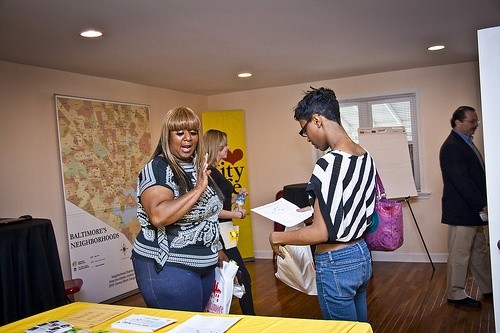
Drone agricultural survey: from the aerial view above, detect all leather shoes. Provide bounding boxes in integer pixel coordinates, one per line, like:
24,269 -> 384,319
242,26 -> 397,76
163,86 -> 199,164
447,296 -> 482,307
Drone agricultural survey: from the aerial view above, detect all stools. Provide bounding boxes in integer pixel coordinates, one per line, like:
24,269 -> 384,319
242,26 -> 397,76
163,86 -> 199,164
63,278 -> 83,303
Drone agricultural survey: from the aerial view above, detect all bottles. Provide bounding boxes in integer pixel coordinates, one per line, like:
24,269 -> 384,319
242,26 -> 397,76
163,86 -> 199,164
235,193 -> 246,212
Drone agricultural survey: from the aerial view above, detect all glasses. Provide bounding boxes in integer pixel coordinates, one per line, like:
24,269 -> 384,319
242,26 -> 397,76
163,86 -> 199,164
299,112 -> 321,137
464,119 -> 480,123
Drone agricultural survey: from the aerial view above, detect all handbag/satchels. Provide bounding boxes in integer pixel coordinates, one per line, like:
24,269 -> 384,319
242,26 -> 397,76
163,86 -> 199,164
274,222 -> 318,295
218,220 -> 239,248
360,170 -> 405,251
203,259 -> 239,314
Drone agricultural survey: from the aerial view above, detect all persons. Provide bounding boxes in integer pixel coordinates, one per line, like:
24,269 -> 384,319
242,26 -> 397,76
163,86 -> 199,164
439,105 -> 493,308
130,105 -> 230,313
269,86 -> 378,323
197,129 -> 257,316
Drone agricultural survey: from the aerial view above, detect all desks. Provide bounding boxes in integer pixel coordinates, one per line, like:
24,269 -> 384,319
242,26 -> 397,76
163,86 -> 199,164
0,301 -> 373,333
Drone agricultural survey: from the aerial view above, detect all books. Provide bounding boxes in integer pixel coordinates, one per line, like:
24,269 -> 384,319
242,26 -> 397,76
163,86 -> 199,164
110,314 -> 177,332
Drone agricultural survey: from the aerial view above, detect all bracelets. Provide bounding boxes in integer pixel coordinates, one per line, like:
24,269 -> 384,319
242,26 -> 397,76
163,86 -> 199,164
239,210 -> 243,219
